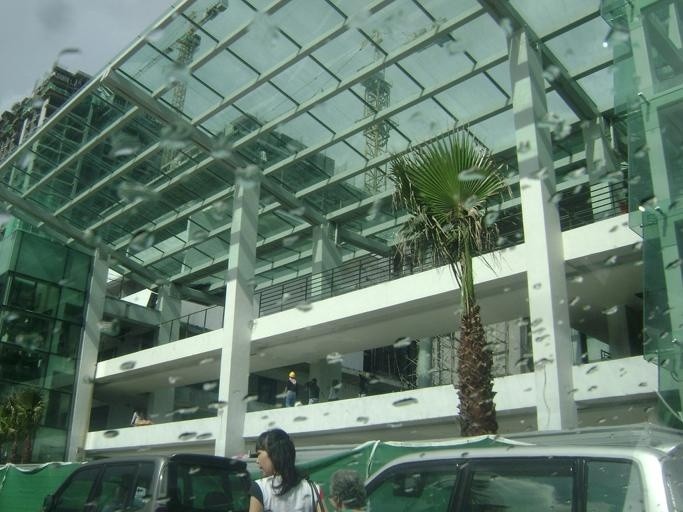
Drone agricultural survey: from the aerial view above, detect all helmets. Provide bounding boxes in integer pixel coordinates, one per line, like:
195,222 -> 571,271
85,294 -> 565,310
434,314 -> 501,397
289,371 -> 296,378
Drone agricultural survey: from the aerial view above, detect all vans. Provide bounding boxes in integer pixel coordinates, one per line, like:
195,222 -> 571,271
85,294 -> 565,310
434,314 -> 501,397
41,451 -> 251,512
358,445 -> 680,512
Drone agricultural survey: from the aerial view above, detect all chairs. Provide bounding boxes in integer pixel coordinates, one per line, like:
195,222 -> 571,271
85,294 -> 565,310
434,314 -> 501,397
203,492 -> 227,512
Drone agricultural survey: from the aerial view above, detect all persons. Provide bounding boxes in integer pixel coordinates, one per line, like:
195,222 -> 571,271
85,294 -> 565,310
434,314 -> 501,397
303,377 -> 320,404
247,429 -> 328,512
327,468 -> 368,512
327,379 -> 340,401
285,370 -> 298,407
131,406 -> 144,426
